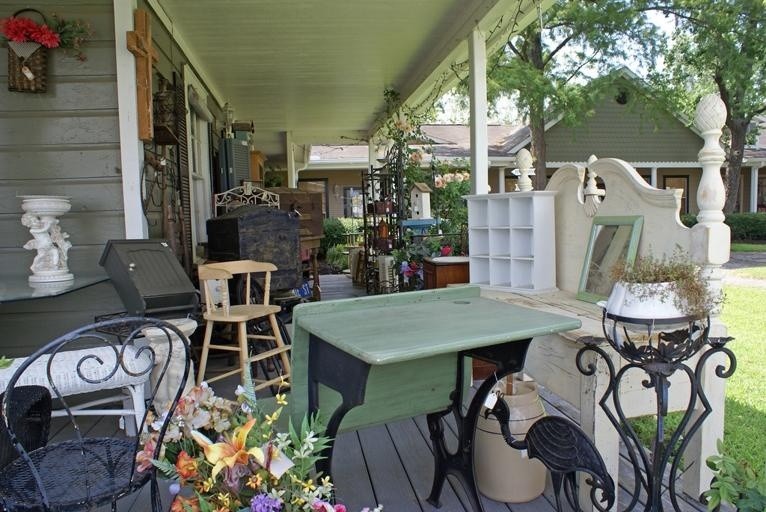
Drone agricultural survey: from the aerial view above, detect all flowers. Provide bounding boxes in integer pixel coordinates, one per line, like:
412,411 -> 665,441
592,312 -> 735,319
0,16 -> 99,65
129,344 -> 387,512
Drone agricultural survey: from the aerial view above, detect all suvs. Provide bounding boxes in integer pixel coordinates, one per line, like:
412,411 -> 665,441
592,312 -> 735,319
474,380 -> 548,503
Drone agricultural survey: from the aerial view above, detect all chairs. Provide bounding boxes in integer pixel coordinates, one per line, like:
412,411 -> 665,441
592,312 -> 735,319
0,317 -> 191,511
196,259 -> 292,412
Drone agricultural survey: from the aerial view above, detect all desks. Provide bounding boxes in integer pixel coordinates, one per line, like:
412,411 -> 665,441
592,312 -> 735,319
289,283 -> 583,511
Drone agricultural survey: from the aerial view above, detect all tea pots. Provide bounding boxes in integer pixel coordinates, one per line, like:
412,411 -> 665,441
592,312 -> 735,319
576,214 -> 645,305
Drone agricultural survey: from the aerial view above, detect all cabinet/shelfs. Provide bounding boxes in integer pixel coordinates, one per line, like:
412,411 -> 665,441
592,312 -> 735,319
460,190 -> 562,296
421,257 -> 469,289
361,164 -> 403,295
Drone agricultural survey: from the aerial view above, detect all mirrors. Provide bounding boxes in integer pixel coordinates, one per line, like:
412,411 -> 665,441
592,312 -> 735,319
576,214 -> 645,305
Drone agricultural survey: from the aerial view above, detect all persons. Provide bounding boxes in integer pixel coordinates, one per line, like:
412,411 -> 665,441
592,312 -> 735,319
21,212 -> 59,272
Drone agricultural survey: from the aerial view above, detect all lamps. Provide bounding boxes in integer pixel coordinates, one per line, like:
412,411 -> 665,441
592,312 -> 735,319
196,259 -> 292,412
0,317 -> 191,511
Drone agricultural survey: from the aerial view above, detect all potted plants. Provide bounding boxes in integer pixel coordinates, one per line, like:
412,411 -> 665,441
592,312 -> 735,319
608,240 -> 731,335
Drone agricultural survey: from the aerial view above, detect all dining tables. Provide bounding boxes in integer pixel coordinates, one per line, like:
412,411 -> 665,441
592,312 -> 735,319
608,240 -> 731,335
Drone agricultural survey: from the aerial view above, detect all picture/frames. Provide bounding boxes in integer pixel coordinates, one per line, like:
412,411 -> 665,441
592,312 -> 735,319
289,283 -> 583,511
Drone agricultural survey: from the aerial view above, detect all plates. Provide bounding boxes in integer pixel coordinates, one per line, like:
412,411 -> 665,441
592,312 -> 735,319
421,257 -> 469,289
361,164 -> 403,295
460,190 -> 562,296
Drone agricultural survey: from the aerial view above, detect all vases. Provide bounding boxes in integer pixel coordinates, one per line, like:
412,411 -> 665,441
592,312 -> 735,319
0,16 -> 99,65
